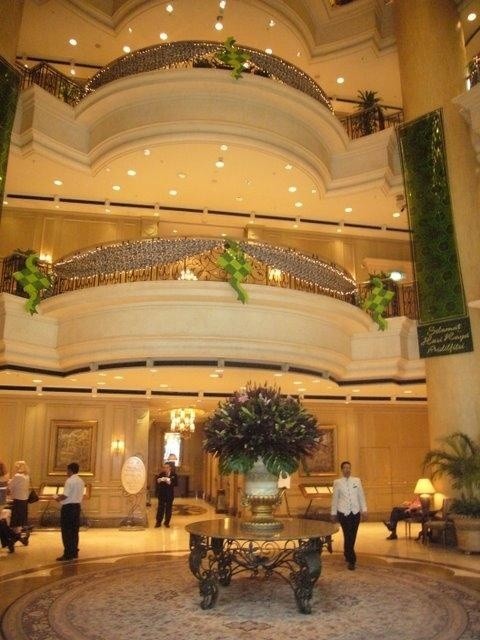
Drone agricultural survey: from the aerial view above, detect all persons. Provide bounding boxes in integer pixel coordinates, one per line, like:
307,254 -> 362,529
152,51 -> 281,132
0,462 -> 10,487
4,460 -> 32,531
329,461 -> 368,571
52,463 -> 85,561
382,496 -> 424,541
153,461 -> 178,528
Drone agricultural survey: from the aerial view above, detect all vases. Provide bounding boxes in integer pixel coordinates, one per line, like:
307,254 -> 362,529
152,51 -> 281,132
237,455 -> 285,534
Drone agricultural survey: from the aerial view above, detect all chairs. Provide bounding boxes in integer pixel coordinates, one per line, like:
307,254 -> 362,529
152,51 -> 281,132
402,491 -> 445,541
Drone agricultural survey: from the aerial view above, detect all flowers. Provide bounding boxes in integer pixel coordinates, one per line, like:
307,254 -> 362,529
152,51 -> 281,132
199,377 -> 326,482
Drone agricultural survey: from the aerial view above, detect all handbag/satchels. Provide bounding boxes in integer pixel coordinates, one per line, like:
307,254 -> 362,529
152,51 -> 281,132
27,491 -> 40,503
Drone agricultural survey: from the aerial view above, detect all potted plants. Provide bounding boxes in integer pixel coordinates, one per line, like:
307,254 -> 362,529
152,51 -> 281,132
420,430 -> 480,556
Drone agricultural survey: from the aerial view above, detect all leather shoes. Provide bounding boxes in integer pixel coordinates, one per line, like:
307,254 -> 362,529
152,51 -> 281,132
73,552 -> 78,558
56,554 -> 73,561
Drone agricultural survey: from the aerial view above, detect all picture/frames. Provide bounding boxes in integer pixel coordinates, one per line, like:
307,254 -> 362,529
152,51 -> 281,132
295,422 -> 342,479
47,417 -> 99,478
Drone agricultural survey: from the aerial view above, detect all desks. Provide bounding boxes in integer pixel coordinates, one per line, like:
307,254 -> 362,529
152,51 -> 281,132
418,516 -> 458,549
184,513 -> 342,615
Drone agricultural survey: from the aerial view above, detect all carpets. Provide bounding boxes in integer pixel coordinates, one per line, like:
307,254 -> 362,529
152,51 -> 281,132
0,554 -> 480,640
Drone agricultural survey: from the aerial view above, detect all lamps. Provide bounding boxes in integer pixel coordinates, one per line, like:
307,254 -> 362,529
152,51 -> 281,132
168,407 -> 198,441
412,479 -> 442,521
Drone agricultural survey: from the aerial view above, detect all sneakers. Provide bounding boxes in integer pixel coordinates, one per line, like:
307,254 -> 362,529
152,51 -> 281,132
155,521 -> 161,527
384,521 -> 394,530
345,552 -> 356,570
386,533 -> 397,539
23,532 -> 30,546
164,521 -> 169,527
